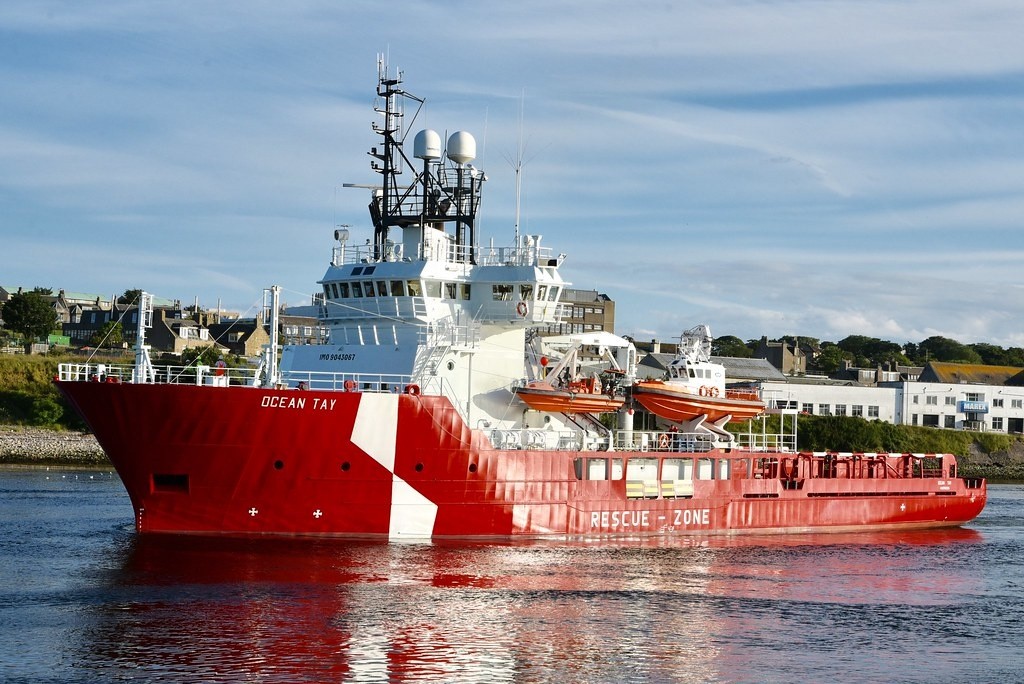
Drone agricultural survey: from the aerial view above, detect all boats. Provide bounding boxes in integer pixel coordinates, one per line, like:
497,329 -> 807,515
626,323 -> 768,425
513,340 -> 627,415
51,42 -> 989,543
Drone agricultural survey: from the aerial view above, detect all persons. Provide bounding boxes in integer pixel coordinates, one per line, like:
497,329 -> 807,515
183,359 -> 195,383
367,284 -> 415,297
667,424 -> 679,447
215,354 -> 227,377
194,356 -> 203,384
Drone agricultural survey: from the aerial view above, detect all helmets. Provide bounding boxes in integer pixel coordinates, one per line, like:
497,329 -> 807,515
219,355 -> 224,360
197,356 -> 201,360
186,359 -> 190,363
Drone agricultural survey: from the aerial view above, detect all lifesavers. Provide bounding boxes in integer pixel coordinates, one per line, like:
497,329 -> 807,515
659,434 -> 668,448
709,387 -> 719,398
699,386 -> 708,396
517,302 -> 528,317
